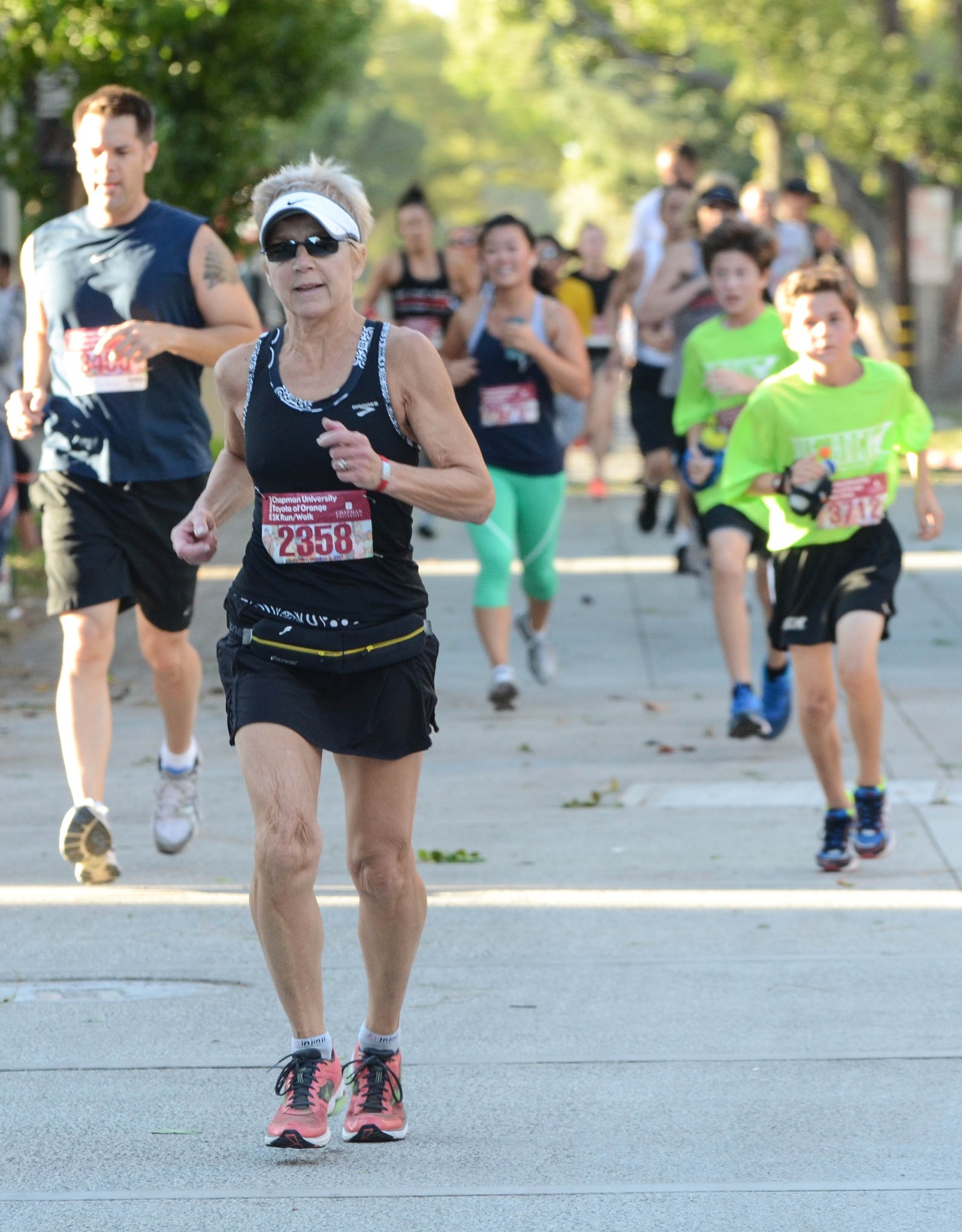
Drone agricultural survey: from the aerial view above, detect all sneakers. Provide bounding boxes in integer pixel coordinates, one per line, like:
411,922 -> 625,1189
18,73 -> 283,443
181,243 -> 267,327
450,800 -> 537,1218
729,686 -> 770,738
488,669 -> 519,711
153,753 -> 198,855
817,790 -> 857,868
517,612 -> 559,685
854,780 -> 889,857
60,803 -> 119,884
339,1044 -> 409,1142
760,659 -> 792,739
265,1047 -> 348,1150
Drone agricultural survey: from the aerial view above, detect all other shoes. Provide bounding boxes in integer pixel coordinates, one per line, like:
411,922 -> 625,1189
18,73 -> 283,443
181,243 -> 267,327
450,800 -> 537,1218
638,489 -> 660,530
588,480 -> 605,498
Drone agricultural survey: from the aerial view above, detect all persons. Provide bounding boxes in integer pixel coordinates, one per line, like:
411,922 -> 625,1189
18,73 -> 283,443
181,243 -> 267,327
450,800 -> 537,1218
4,84 -> 263,884
363,182 -> 472,538
170,151 -> 495,1147
569,221 -> 623,500
437,213 -> 592,710
637,182 -> 757,561
446,139 -> 867,575
0,213 -> 286,636
672,220 -> 798,741
719,262 -> 944,872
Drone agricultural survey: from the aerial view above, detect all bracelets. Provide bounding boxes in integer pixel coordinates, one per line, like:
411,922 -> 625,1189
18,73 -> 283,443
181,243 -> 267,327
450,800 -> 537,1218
375,454 -> 391,492
771,467 -> 793,496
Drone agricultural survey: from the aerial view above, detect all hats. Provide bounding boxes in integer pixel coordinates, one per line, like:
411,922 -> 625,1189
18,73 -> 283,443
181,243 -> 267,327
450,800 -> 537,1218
786,180 -> 821,206
694,186 -> 739,211
259,193 -> 361,247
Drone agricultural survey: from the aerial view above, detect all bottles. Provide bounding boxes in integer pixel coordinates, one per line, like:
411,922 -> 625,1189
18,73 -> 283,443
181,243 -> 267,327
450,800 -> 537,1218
789,448 -> 832,511
503,317 -> 525,370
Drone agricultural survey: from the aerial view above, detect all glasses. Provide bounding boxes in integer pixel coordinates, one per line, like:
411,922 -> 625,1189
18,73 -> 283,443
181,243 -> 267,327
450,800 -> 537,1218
262,236 -> 346,261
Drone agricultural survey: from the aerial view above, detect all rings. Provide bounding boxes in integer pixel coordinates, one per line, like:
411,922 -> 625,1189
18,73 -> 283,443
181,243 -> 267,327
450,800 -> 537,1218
338,457 -> 346,470
